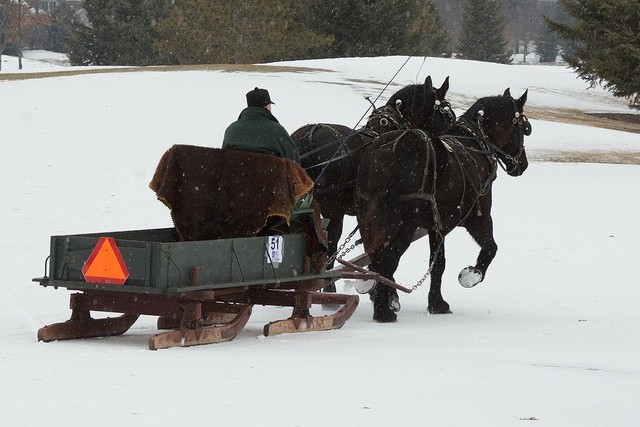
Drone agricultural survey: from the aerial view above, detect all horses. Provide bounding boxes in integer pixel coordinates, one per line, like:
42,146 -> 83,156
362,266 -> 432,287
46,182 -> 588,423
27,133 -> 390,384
356,87 -> 529,322
290,74 -> 456,311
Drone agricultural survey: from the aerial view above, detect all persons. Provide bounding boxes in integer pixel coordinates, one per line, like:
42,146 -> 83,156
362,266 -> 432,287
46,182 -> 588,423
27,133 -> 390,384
221,87 -> 299,168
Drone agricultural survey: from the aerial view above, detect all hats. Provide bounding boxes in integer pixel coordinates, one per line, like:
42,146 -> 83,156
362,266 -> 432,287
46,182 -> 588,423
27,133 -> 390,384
246,86 -> 275,107
